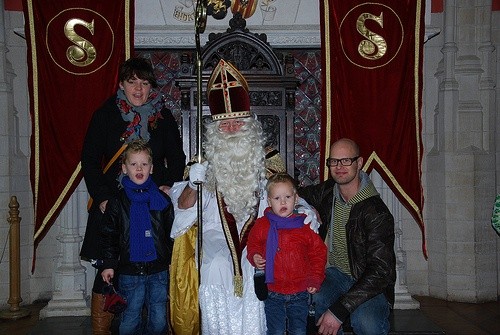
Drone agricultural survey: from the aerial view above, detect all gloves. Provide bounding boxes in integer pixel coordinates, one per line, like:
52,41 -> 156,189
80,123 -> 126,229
189,161 -> 209,191
297,209 -> 321,235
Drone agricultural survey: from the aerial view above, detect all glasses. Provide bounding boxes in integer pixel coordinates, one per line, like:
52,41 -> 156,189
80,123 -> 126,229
326,156 -> 359,166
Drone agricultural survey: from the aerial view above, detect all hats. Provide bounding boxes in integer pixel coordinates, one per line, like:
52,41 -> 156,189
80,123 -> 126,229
206,59 -> 251,121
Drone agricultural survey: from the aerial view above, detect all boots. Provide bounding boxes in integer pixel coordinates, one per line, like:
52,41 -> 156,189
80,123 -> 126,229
91,288 -> 114,335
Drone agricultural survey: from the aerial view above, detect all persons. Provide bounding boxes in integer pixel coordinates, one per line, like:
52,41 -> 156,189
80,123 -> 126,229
297,138 -> 396,335
98,140 -> 175,335
244,172 -> 329,335
169,58 -> 322,335
80,55 -> 187,335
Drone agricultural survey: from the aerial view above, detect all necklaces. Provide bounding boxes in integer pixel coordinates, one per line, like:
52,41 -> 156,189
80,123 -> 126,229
339,192 -> 347,204
